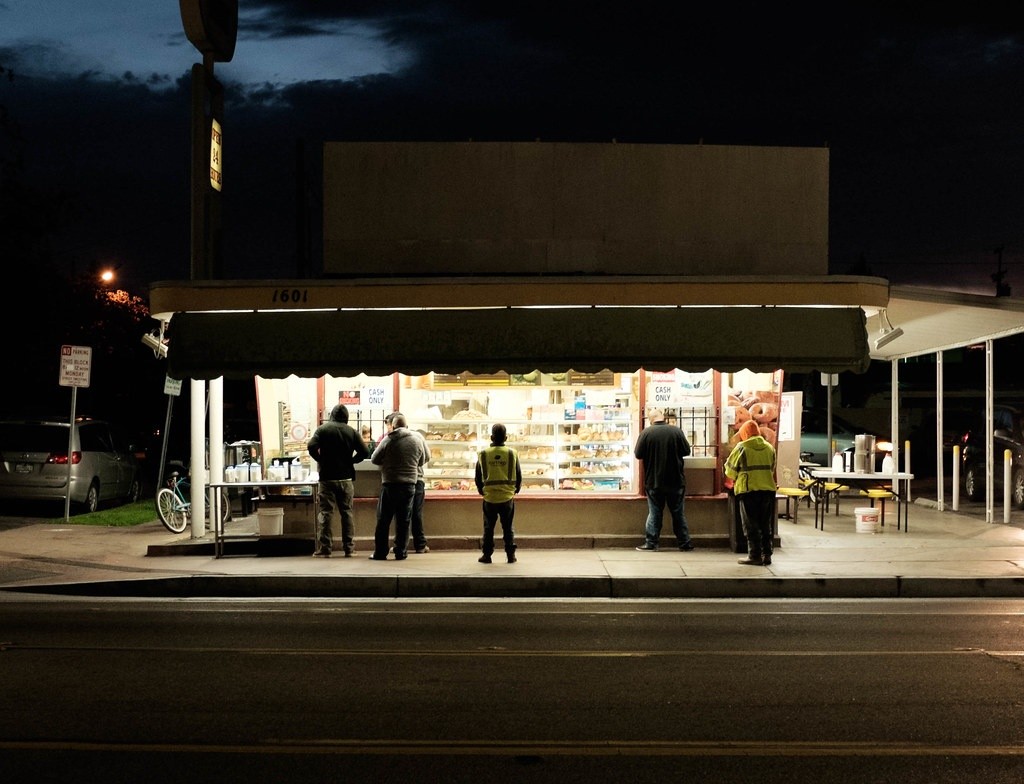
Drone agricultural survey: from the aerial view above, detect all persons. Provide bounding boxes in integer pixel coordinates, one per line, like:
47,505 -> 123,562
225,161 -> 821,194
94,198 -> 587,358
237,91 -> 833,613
475,423 -> 522,563
635,408 -> 696,552
369,415 -> 426,561
307,404 -> 369,558
361,415 -> 393,459
389,412 -> 430,553
724,420 -> 777,566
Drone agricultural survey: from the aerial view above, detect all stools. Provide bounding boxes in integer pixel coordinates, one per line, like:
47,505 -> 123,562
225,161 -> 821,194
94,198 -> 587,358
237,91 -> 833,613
777,478 -> 893,526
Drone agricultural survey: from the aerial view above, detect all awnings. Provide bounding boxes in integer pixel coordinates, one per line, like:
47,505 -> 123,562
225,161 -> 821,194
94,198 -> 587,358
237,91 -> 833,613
164,307 -> 870,380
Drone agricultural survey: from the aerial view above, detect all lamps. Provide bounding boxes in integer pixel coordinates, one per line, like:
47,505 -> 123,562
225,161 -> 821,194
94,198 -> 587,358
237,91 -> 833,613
874,310 -> 904,350
142,320 -> 168,358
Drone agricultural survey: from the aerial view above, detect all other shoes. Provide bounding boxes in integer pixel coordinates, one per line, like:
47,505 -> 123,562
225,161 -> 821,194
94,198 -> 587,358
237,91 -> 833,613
345,551 -> 358,557
390,547 -> 394,553
507,555 -> 517,563
636,543 -> 658,552
679,546 -> 695,552
738,555 -> 771,566
478,555 -> 492,563
416,546 -> 430,552
313,551 -> 332,558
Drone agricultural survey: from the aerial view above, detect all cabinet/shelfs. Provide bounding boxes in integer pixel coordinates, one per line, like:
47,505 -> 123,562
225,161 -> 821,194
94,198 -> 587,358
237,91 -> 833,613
410,420 -> 632,495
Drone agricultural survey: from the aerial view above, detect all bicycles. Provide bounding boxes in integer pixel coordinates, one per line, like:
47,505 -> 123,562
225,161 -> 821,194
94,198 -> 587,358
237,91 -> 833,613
153,460 -> 230,536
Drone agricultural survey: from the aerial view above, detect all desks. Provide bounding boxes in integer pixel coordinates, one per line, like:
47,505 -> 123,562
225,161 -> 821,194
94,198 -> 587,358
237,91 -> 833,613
210,479 -> 320,559
799,463 -> 914,533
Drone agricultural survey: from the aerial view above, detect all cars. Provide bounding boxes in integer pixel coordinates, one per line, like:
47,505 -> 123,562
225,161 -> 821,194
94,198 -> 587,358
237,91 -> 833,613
0,413 -> 145,513
943,430 -> 961,454
800,407 -> 899,470
961,399 -> 1024,507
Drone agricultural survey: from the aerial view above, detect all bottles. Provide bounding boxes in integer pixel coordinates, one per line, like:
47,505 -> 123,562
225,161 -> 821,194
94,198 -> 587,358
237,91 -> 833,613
832,452 -> 843,473
226,462 -> 303,483
882,453 -> 895,476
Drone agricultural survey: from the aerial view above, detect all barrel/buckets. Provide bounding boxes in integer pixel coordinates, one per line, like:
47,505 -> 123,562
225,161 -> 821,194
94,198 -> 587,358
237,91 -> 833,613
854,507 -> 879,533
257,507 -> 284,535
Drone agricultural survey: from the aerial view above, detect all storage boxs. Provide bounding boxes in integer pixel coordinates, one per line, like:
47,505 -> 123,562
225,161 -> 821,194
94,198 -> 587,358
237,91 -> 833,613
595,480 -> 619,490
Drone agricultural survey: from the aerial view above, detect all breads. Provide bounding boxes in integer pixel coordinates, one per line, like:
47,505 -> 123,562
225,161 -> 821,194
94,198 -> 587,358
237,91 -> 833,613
417,429 -> 555,490
728,391 -> 779,447
560,428 -> 629,490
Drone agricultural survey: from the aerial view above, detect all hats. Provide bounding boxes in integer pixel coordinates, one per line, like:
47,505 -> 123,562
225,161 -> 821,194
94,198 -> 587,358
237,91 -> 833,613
648,407 -> 665,422
